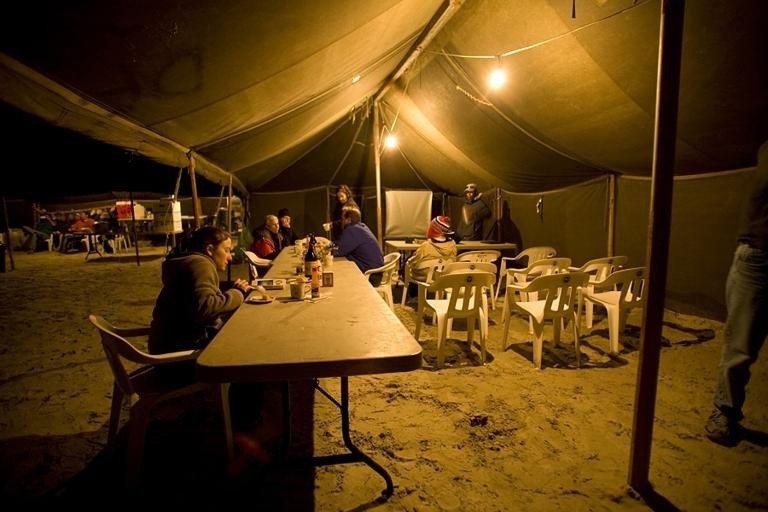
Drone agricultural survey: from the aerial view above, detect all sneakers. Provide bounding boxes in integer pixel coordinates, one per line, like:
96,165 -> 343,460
705,409 -> 734,442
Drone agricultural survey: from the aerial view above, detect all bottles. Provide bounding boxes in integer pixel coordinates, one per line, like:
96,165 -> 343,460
312,266 -> 320,298
304,243 -> 318,280
310,233 -> 317,243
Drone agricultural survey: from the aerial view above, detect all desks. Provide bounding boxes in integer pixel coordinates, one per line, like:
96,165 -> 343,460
385,238 -> 516,251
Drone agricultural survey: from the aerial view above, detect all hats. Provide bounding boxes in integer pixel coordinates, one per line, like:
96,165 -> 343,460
278,208 -> 291,218
426,216 -> 455,237
464,183 -> 476,192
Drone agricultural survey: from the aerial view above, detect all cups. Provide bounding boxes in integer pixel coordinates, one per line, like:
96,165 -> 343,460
290,246 -> 297,257
322,223 -> 331,232
294,240 -> 302,254
289,283 -> 311,298
296,281 -> 305,301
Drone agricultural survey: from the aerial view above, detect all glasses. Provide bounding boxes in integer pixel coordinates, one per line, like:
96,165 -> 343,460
465,187 -> 475,188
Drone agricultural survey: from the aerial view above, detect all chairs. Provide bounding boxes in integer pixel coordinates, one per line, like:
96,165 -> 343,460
46,230 -> 132,254
402,246 -> 650,369
364,251 -> 401,313
89,314 -> 240,480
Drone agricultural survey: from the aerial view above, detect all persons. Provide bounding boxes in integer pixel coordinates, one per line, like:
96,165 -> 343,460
331,209 -> 384,288
703,145 -> 768,440
333,184 -> 360,231
250,214 -> 283,279
406,216 -> 457,304
456,183 -> 492,241
278,208 -> 303,246
147,227 -> 264,437
24,212 -> 134,254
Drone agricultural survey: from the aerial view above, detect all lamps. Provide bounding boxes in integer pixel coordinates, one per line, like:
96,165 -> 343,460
489,53 -> 507,93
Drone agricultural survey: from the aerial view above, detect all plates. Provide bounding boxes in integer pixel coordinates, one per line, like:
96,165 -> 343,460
247,296 -> 275,303
